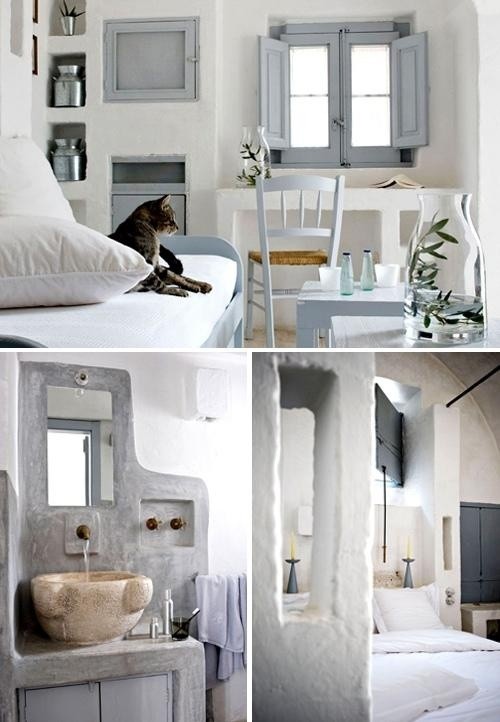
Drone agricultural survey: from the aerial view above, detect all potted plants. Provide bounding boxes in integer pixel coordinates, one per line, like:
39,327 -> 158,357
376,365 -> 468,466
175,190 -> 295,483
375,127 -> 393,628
58,1 -> 84,36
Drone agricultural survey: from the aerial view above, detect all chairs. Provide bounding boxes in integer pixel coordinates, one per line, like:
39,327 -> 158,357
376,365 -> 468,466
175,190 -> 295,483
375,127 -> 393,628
246,173 -> 345,347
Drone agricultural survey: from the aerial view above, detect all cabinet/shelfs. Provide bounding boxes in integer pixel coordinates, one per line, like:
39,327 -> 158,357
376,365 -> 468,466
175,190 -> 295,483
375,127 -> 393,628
460,500 -> 500,604
18,672 -> 174,721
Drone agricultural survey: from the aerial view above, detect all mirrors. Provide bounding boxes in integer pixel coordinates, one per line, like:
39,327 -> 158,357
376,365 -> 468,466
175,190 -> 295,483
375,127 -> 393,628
45,384 -> 114,508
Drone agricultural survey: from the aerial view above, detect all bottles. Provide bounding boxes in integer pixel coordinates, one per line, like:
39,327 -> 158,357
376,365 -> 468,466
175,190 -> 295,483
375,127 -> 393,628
149,588 -> 173,639
340,252 -> 355,294
360,249 -> 376,289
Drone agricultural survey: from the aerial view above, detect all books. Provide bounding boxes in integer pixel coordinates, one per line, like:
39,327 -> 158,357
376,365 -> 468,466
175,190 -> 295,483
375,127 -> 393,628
374,174 -> 422,189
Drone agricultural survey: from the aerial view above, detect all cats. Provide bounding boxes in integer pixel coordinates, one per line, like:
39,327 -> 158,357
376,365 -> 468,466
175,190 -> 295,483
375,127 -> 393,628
107,194 -> 212,298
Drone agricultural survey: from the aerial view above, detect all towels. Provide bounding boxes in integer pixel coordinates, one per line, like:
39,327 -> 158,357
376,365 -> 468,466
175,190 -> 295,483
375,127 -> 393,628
195,573 -> 247,690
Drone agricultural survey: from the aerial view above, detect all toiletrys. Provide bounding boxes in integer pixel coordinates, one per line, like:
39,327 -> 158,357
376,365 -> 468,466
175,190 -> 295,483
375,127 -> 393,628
151,616 -> 158,639
161,589 -> 175,635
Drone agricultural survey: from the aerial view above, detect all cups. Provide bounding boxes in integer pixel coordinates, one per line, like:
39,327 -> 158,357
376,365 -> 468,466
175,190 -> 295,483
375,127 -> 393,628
374,264 -> 401,288
60,17 -> 76,36
170,618 -> 190,640
318,266 -> 340,291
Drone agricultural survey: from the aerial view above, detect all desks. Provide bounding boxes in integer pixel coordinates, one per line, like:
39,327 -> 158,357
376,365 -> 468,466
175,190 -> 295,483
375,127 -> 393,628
215,186 -> 462,281
330,315 -> 499,347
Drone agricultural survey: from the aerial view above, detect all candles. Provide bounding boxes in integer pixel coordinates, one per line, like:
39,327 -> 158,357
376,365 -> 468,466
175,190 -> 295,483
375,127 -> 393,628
407,536 -> 412,558
290,532 -> 296,560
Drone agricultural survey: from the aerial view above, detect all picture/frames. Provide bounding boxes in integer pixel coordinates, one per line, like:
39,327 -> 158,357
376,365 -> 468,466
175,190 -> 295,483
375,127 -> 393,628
32,0 -> 38,23
33,34 -> 38,74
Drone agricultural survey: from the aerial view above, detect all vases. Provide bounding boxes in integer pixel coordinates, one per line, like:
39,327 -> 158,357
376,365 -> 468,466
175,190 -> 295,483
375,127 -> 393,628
404,192 -> 486,344
236,125 -> 271,187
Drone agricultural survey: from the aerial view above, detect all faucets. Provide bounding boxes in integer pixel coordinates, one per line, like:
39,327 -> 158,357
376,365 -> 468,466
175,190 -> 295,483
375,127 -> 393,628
75,525 -> 91,540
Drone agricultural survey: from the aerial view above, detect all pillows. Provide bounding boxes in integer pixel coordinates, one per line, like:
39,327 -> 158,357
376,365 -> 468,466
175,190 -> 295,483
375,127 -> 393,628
1,137 -> 77,223
372,581 -> 454,633
0,215 -> 155,310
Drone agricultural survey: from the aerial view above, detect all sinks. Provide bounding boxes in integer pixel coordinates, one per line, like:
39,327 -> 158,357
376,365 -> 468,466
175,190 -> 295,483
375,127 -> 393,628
31,571 -> 154,645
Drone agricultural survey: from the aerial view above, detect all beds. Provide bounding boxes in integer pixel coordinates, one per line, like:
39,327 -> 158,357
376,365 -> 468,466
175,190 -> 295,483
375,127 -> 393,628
0,233 -> 245,347
372,570 -> 500,720
297,280 -> 406,346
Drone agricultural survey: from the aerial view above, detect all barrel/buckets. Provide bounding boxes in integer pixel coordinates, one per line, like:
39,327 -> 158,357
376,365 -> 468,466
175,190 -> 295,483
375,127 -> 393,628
52,64 -> 87,106
49,136 -> 85,181
